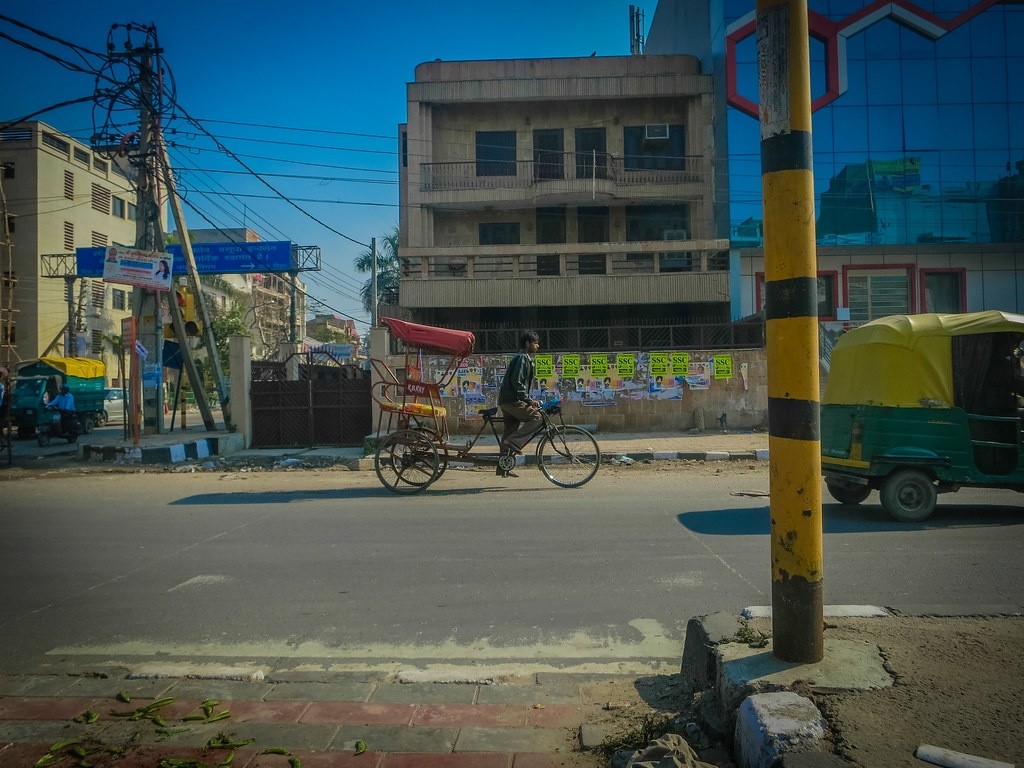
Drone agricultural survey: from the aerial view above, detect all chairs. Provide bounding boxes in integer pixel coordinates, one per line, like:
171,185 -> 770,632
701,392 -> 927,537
368,358 -> 449,445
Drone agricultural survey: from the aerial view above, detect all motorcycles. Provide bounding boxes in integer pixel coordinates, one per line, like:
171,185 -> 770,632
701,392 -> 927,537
11,357 -> 106,438
36,405 -> 79,446
820,309 -> 1023,521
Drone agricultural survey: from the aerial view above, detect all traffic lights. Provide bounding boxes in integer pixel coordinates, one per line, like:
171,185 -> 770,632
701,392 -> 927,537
164,279 -> 204,339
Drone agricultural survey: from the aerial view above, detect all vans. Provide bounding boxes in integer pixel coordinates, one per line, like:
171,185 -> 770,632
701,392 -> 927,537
97,385 -> 131,427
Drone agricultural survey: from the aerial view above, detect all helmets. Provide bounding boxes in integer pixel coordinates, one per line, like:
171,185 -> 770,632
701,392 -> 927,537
60,384 -> 69,392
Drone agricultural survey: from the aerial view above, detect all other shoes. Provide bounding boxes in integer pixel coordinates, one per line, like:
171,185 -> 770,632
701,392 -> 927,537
495,471 -> 519,477
502,439 -> 522,455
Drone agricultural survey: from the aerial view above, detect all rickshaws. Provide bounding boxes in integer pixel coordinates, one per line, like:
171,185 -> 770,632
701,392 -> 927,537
369,315 -> 602,494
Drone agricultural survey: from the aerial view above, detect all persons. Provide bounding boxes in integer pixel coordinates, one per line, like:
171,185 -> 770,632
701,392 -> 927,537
603,377 -> 613,389
540,378 -> 547,391
698,366 -> 707,380
154,260 -> 170,280
577,378 -> 585,390
654,376 -> 665,389
462,380 -> 469,393
496,330 -> 543,478
46,383 -> 76,435
